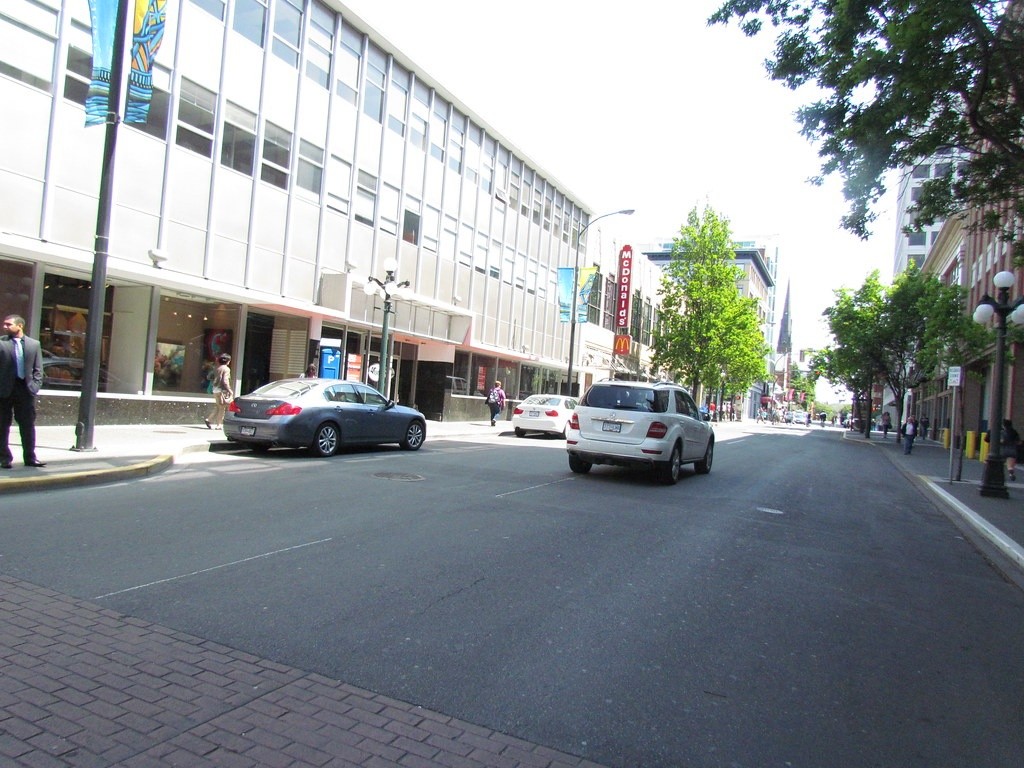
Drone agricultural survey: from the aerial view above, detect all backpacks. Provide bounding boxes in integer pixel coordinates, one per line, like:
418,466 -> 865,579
488,389 -> 499,403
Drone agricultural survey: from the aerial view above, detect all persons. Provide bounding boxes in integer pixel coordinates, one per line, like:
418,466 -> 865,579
298,364 -> 318,378
205,353 -> 234,430
0,314 -> 46,467
487,381 -> 505,427
706,401 -> 845,428
871,411 -> 930,455
985,419 -> 1022,481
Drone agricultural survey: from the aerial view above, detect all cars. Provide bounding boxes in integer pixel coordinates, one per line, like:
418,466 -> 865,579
783,410 -> 809,426
221,377 -> 427,458
511,394 -> 579,440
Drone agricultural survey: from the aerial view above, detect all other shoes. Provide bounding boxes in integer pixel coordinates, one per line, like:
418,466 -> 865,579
25,459 -> 46,466
1008,468 -> 1016,482
204,419 -> 211,429
215,427 -> 222,430
0,459 -> 13,468
491,420 -> 497,426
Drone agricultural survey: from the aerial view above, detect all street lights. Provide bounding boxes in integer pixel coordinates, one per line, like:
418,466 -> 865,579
567,209 -> 636,394
365,255 -> 416,396
972,270 -> 1024,501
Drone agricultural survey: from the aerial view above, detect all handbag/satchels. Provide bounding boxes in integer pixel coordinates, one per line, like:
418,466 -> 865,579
888,423 -> 892,429
220,389 -> 234,405
206,370 -> 214,395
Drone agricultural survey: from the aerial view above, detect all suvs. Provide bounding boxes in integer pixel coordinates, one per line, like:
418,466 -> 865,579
566,377 -> 715,486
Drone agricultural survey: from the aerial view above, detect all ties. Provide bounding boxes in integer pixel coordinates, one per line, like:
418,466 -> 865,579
13,337 -> 25,380
